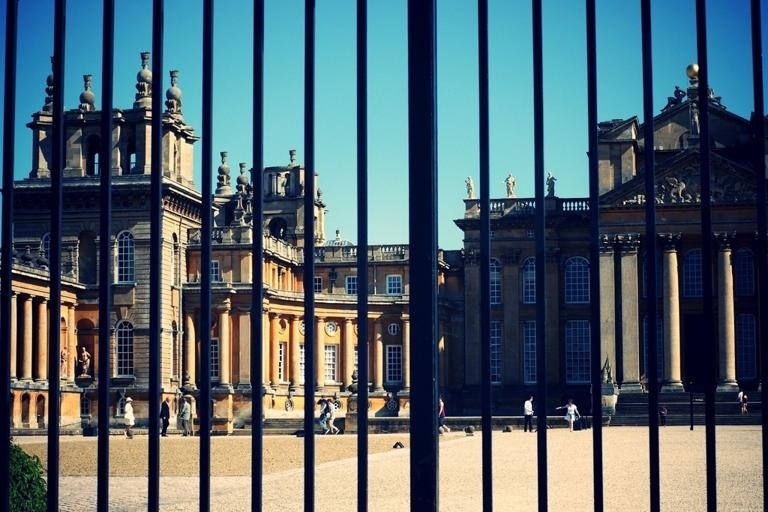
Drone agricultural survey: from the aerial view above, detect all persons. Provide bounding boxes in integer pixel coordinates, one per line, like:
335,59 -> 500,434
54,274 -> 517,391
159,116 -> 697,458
660,85 -> 726,136
123,397 -> 135,439
736,389 -> 749,415
61,347 -> 91,379
546,171 -> 557,197
504,173 -> 516,198
555,398 -> 580,432
316,398 -> 340,435
160,397 -> 192,438
465,176 -> 474,198
659,406 -> 667,428
438,393 -> 451,434
523,394 -> 535,433
